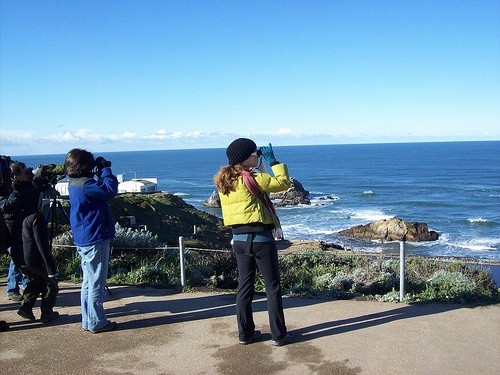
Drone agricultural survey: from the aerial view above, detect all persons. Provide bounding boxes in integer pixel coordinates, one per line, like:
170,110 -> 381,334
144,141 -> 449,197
0,155 -> 58,330
65,149 -> 118,333
215,138 -> 292,347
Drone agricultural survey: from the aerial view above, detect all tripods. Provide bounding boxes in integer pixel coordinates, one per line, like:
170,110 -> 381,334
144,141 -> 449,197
45,186 -> 112,295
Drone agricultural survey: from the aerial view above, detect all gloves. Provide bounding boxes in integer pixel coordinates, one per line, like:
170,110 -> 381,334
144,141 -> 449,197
261,143 -> 275,164
47,273 -> 59,284
95,156 -> 107,169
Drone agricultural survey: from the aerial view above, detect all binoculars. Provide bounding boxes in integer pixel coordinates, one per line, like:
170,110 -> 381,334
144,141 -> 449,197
258,150 -> 264,157
94,158 -> 112,170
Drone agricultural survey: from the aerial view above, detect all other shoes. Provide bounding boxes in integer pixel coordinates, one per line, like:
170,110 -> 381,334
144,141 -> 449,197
272,333 -> 295,346
90,320 -> 116,334
17,307 -> 36,321
42,311 -> 60,323
239,330 -> 261,344
7,292 -> 23,301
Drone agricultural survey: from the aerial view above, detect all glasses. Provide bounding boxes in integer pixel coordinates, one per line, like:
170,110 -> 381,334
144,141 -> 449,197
251,150 -> 260,158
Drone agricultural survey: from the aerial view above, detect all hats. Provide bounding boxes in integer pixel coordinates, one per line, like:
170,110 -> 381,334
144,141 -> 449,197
225,138 -> 257,165
